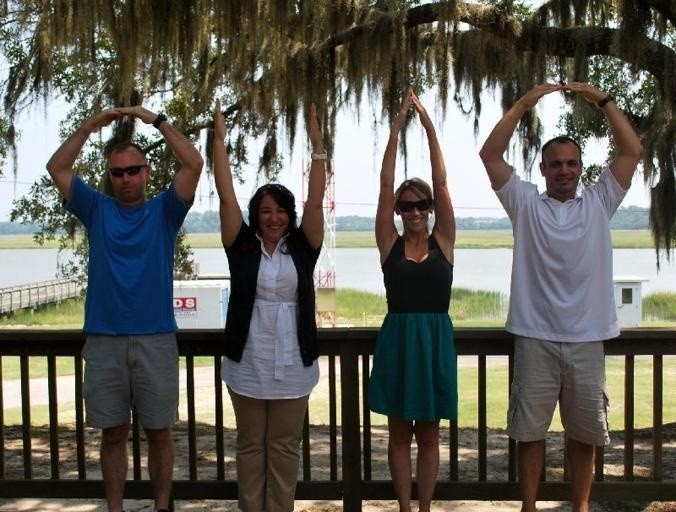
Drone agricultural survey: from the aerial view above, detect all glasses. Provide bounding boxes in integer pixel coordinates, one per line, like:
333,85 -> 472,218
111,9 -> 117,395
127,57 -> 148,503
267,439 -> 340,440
398,199 -> 432,212
109,165 -> 148,177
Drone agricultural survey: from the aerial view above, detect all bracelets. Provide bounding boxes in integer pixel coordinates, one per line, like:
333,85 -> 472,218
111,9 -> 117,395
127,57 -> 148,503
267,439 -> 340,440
312,153 -> 327,160
598,94 -> 615,108
152,114 -> 166,128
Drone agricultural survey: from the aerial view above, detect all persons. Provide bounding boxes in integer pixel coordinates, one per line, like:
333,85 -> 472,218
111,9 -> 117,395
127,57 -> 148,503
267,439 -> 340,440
479,81 -> 641,512
214,98 -> 327,512
46,105 -> 204,512
368,87 -> 457,512
